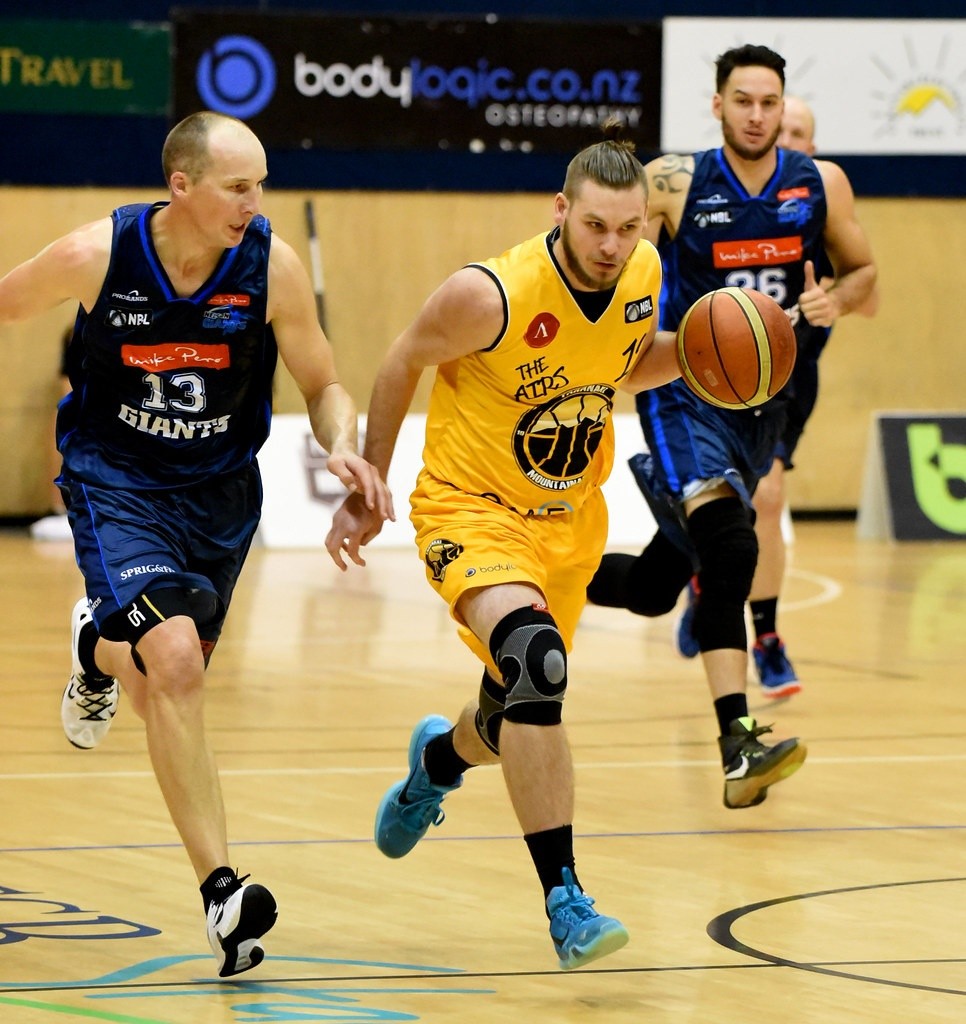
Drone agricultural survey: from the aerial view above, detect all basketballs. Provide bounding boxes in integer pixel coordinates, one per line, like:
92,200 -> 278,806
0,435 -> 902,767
675,286 -> 799,409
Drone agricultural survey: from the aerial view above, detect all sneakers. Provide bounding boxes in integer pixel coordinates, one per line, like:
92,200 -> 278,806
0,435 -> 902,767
718,716 -> 807,809
546,868 -> 629,969
205,868 -> 278,977
63,597 -> 124,750
750,629 -> 801,696
376,713 -> 471,859
674,577 -> 703,657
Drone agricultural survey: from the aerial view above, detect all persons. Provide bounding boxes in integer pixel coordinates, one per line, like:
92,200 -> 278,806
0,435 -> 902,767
673,97 -> 877,699
323,117 -> 684,970
0,111 -> 395,977
587,43 -> 877,807
